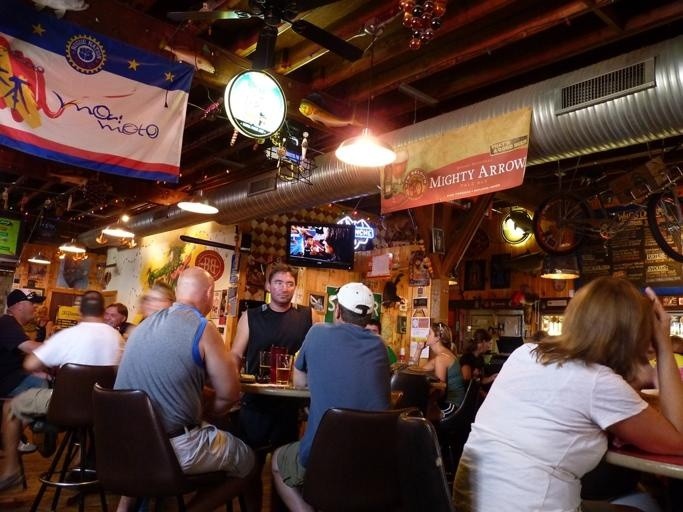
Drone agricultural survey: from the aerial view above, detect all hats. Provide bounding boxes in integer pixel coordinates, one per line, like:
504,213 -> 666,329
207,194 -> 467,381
336,282 -> 374,315
7,288 -> 46,308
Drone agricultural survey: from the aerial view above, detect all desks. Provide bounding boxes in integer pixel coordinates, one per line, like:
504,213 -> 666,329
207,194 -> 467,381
595,447 -> 680,479
238,381 -> 313,404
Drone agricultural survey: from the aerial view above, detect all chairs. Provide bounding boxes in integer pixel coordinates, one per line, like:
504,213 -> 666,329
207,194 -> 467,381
441,379 -> 477,478
30,360 -> 119,512
86,379 -> 238,512
389,370 -> 428,417
290,406 -> 457,509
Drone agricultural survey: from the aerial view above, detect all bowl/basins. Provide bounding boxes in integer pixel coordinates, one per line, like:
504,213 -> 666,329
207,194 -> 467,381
390,391 -> 403,409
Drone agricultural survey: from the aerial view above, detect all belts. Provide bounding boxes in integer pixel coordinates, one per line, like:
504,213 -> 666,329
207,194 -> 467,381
169,422 -> 197,437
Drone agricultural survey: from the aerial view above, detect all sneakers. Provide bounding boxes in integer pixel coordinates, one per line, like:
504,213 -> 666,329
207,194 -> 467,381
17,440 -> 37,453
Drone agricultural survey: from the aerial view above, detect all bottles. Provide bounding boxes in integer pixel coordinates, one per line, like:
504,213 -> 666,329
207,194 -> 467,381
399,348 -> 405,362
390,369 -> 400,392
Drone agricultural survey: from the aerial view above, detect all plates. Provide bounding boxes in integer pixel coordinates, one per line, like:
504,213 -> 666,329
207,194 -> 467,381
239,377 -> 255,384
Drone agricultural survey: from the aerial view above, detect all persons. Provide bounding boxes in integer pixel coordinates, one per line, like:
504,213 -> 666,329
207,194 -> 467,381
459,328 -> 499,398
411,321 -> 465,420
229,263 -> 322,378
112,265 -> 261,511
270,282 -> 392,512
365,319 -> 396,364
0,284 -> 175,487
449,275 -> 683,512
290,225 -> 350,263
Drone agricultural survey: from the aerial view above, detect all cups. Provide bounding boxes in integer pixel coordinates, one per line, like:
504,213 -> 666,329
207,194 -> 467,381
275,353 -> 292,388
258,351 -> 271,383
408,359 -> 417,369
270,346 -> 288,387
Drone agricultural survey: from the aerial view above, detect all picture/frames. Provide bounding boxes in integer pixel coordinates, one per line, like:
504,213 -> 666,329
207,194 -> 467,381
301,288 -> 328,316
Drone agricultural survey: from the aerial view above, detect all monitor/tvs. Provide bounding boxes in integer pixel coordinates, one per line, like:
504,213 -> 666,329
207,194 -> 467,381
286,220 -> 356,270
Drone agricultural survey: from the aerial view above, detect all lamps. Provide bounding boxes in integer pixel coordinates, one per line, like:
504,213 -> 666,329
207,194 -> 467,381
172,188 -> 221,215
26,248 -> 53,266
538,262 -> 581,282
101,210 -> 135,243
332,2 -> 398,169
56,237 -> 87,254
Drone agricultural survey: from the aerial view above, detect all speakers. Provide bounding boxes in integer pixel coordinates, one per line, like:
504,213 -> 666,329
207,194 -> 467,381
240,233 -> 252,251
